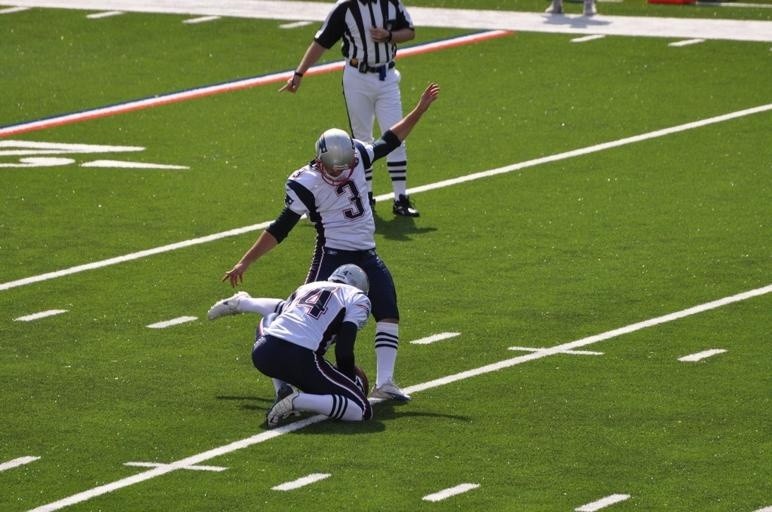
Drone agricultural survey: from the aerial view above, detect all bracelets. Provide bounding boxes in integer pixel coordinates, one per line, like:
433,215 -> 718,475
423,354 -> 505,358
388,31 -> 393,42
293,72 -> 305,77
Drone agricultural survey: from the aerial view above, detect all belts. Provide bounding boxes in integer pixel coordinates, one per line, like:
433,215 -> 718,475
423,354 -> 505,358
350,59 -> 394,72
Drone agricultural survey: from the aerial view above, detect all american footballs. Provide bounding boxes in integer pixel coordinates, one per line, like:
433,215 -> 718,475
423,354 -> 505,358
338,365 -> 370,397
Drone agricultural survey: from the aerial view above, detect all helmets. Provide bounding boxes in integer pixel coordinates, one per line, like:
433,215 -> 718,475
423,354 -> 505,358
315,128 -> 355,186
327,263 -> 370,296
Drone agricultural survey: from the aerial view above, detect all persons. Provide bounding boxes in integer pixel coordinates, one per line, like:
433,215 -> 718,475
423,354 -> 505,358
251,264 -> 374,426
544,0 -> 596,17
276,1 -> 421,217
207,83 -> 440,400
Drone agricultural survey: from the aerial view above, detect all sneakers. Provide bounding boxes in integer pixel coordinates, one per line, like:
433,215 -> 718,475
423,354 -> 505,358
266,391 -> 300,428
583,1 -> 597,15
370,382 -> 411,401
393,194 -> 420,216
545,3 -> 563,12
278,385 -> 302,424
207,290 -> 251,321
